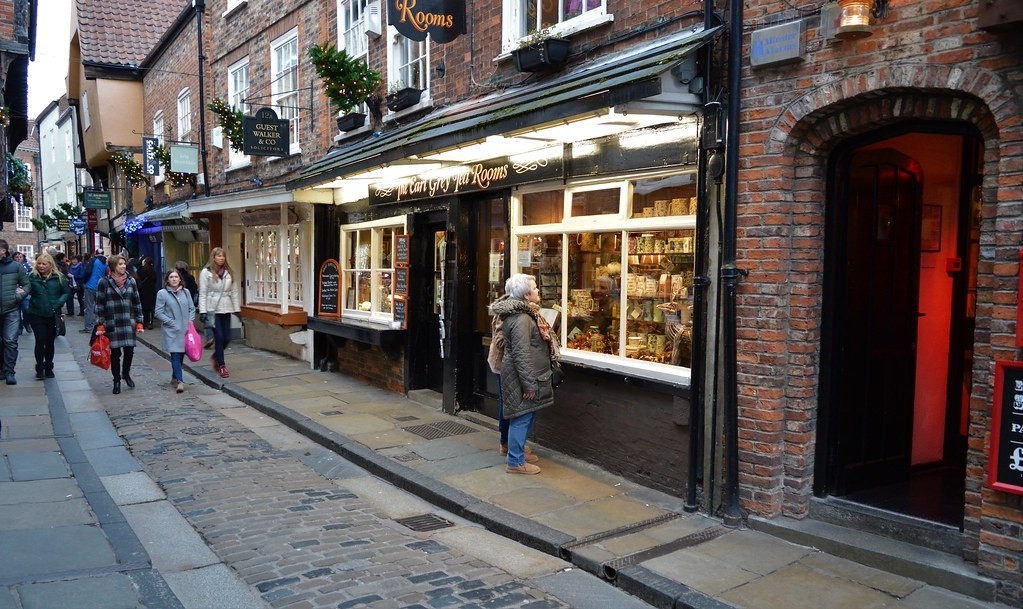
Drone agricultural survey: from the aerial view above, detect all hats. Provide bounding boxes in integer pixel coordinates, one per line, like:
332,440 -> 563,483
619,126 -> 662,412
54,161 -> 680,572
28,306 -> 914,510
175,261 -> 188,271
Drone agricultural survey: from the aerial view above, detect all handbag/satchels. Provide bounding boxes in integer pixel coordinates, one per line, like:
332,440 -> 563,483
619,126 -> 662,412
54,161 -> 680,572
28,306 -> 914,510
193,291 -> 198,307
54,312 -> 65,336
89,324 -> 110,347
91,335 -> 111,370
203,311 -> 216,329
184,321 -> 202,362
69,274 -> 77,289
549,366 -> 566,388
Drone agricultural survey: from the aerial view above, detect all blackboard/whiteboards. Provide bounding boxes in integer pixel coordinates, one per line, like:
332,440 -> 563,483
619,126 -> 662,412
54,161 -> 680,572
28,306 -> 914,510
394,234 -> 410,265
393,297 -> 410,329
392,267 -> 409,295
318,259 -> 342,316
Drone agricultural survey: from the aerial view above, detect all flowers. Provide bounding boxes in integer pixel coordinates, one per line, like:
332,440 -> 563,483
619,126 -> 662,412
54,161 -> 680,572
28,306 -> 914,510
384,79 -> 404,109
518,22 -> 563,62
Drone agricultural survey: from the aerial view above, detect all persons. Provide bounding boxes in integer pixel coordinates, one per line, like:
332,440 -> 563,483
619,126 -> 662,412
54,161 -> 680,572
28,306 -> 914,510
0,239 -> 198,385
95,255 -> 144,393
487,274 -> 554,475
592,277 -> 624,334
155,269 -> 195,393
198,248 -> 242,378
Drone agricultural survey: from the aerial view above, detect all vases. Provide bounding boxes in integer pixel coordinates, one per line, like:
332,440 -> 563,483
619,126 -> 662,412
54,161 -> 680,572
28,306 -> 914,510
511,37 -> 571,72
336,112 -> 367,131
385,86 -> 421,111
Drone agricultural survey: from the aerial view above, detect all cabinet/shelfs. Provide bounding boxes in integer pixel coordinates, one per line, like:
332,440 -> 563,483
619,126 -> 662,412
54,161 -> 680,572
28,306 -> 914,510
536,249 -> 571,309
609,254 -> 694,334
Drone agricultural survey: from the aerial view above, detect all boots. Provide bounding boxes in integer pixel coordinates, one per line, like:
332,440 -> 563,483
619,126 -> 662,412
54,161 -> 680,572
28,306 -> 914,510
44,362 -> 54,378
36,365 -> 45,379
122,359 -> 135,387
109,360 -> 120,394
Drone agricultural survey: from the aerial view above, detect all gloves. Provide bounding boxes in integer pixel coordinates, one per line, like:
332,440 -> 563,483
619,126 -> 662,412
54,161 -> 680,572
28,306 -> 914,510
234,312 -> 244,327
200,313 -> 209,323
96,323 -> 105,335
136,322 -> 144,334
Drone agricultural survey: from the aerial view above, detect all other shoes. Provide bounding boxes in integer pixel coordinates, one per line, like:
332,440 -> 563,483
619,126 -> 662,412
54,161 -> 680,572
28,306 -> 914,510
77,312 -> 84,316
177,380 -> 183,392
79,329 -> 92,333
66,312 -> 74,315
172,377 -> 178,388
17,319 -> 32,335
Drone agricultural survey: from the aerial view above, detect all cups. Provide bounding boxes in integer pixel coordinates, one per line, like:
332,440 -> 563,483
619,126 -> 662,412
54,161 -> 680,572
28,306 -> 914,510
624,235 -> 693,359
577,296 -> 594,312
571,289 -> 591,309
633,196 -> 697,218
577,232 -> 595,251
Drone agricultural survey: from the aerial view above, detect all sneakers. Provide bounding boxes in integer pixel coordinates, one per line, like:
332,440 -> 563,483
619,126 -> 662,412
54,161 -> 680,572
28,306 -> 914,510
500,442 -> 531,456
0,371 -> 16,384
218,364 -> 229,377
525,454 -> 538,463
506,460 -> 540,475
212,353 -> 219,371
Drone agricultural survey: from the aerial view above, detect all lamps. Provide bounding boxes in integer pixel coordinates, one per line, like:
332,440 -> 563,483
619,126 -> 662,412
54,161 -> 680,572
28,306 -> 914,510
834,0 -> 890,40
